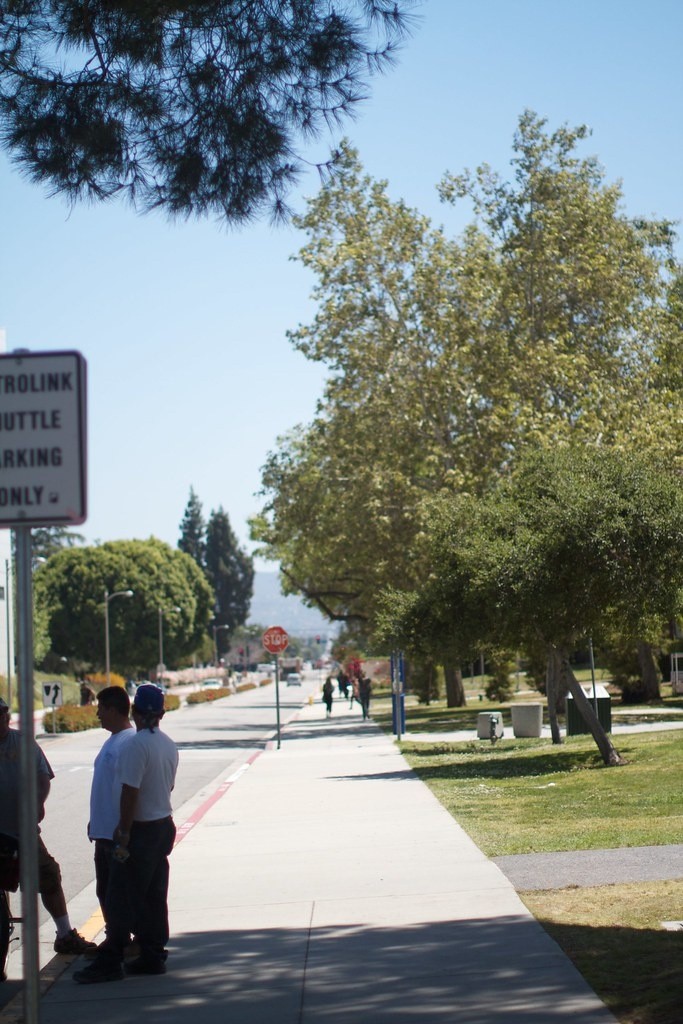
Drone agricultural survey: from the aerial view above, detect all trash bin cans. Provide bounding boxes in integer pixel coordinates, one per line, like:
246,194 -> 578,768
567,684 -> 611,735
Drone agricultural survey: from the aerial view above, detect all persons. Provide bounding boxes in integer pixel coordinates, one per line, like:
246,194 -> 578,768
323,669 -> 372,722
72,680 -> 180,983
0,698 -> 98,956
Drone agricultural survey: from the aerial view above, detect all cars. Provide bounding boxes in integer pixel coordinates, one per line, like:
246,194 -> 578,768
204,679 -> 221,690
287,673 -> 301,687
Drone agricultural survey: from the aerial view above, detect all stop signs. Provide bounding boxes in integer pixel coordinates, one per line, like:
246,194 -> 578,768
263,627 -> 289,655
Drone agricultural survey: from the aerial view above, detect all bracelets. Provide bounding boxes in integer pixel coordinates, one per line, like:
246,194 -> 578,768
119,830 -> 129,838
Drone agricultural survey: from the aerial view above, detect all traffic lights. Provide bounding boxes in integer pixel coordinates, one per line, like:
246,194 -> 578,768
316,635 -> 320,644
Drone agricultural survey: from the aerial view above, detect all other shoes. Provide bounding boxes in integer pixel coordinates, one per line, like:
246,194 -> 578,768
122,943 -> 146,960
84,939 -> 110,959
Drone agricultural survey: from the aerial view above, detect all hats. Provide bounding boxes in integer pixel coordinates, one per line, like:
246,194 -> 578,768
134,684 -> 165,716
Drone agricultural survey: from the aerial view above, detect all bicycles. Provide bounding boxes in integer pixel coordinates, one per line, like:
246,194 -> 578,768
0,846 -> 25,988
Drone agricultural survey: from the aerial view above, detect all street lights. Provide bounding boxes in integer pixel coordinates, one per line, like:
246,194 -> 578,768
157,607 -> 182,693
104,590 -> 133,686
213,625 -> 230,668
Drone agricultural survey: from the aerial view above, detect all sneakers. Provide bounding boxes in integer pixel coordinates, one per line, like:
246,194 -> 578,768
53,928 -> 97,954
72,953 -> 124,984
123,948 -> 167,976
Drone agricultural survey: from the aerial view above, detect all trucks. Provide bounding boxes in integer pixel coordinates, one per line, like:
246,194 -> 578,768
279,658 -> 304,681
